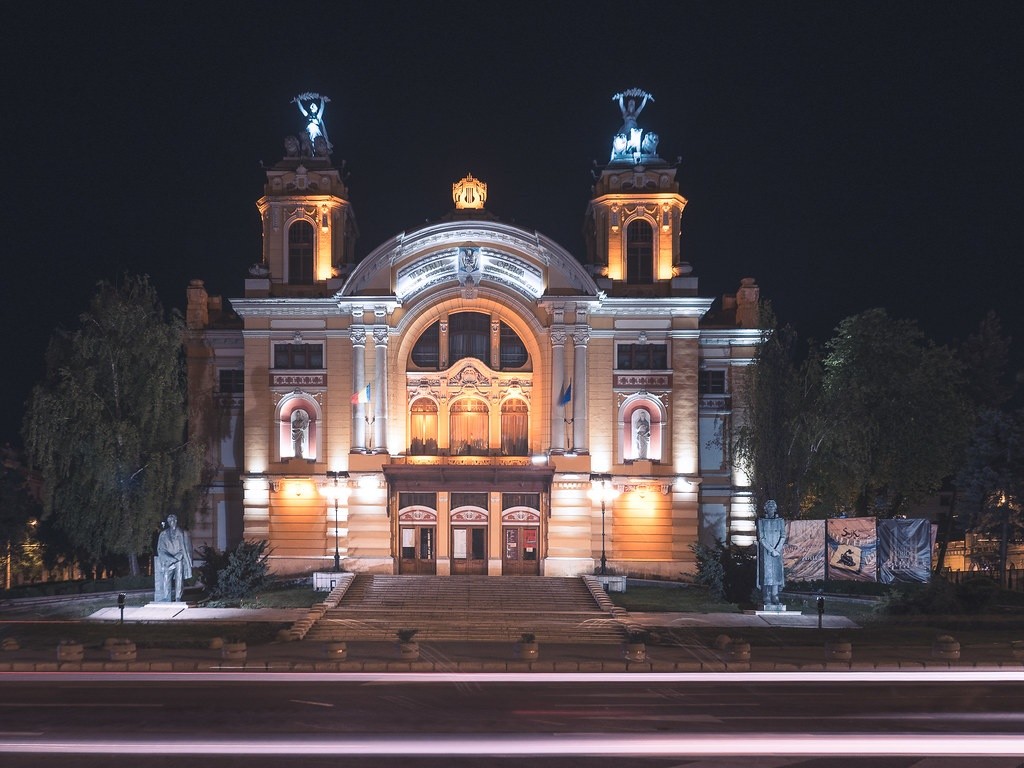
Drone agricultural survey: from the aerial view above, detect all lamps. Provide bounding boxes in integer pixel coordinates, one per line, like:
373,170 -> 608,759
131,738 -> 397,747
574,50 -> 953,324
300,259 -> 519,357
817,596 -> 825,614
117,592 -> 126,609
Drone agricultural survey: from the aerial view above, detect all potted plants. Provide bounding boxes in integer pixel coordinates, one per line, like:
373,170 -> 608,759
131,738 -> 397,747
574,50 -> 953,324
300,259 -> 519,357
621,629 -> 651,664
720,628 -> 780,663
222,634 -> 248,663
519,633 -> 539,663
396,628 -> 419,662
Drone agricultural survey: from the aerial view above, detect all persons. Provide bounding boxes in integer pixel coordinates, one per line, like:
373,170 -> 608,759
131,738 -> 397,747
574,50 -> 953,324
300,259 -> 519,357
618,93 -> 648,153
157,513 -> 194,601
756,500 -> 788,605
297,96 -> 333,152
292,410 -> 308,458
636,412 -> 650,459
460,249 -> 479,273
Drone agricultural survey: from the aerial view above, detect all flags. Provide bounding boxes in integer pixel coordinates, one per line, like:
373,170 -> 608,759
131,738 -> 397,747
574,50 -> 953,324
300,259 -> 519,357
347,384 -> 370,404
559,384 -> 572,406
